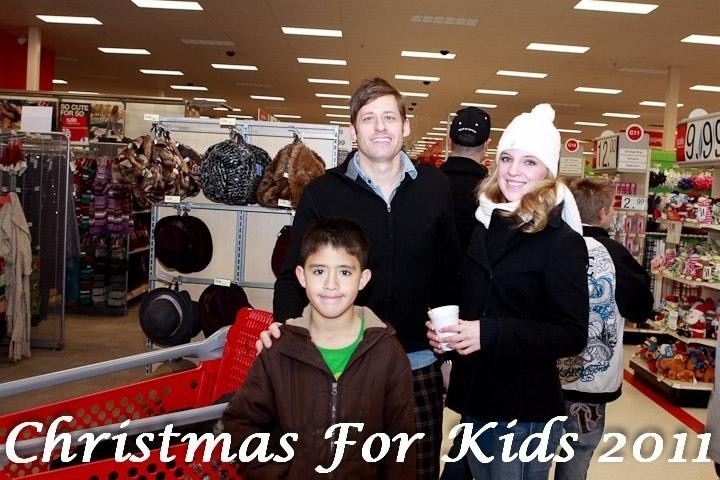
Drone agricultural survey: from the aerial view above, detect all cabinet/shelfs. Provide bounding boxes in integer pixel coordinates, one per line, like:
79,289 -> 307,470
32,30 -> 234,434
592,159 -> 720,408
126,209 -> 151,302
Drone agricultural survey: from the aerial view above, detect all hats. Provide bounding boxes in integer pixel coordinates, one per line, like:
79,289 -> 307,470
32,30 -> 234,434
111,136 -> 327,207
137,279 -> 253,348
495,103 -> 562,180
154,214 -> 213,273
450,107 -> 491,146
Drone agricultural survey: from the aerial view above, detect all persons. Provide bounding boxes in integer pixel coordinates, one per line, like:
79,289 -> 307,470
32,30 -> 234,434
555,173 -> 653,480
425,101 -> 589,480
221,217 -> 420,479
438,107 -> 493,243
255,76 -> 462,477
632,190 -> 719,383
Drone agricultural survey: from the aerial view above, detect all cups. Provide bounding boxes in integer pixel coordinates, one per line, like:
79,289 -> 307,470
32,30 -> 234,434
428,304 -> 460,353
